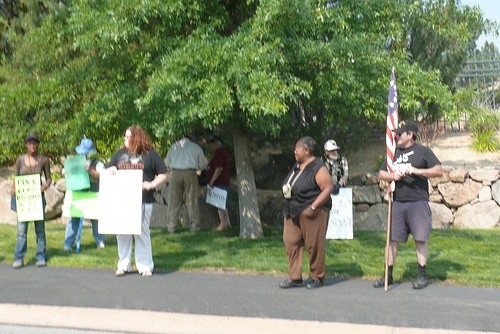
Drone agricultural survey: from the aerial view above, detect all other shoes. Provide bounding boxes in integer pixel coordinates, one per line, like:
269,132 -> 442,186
413,264 -> 428,288
280,279 -> 324,288
166,226 -> 234,233
12,239 -> 153,276
374,266 -> 394,288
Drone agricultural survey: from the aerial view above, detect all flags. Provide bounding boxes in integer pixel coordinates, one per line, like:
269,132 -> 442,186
384,66 -> 399,202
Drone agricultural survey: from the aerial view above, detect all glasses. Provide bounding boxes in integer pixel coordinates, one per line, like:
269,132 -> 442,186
397,132 -> 401,136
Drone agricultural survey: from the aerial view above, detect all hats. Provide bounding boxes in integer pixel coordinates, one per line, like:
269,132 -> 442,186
76,138 -> 96,154
25,134 -> 39,142
324,139 -> 340,150
391,119 -> 418,132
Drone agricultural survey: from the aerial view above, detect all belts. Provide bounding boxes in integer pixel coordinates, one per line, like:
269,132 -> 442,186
173,168 -> 197,170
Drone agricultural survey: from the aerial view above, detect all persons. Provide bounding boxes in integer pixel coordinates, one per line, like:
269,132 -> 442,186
10,134 -> 52,268
319,139 -> 350,188
279,136 -> 335,289
108,124 -> 169,277
163,132 -> 207,235
62,138 -> 105,252
373,121 -> 443,289
207,135 -> 232,231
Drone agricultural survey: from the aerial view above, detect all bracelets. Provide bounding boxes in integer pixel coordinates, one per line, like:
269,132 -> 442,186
311,205 -> 320,213
44,183 -> 48,188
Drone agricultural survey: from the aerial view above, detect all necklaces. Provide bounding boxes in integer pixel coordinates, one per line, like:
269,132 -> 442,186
27,152 -> 37,174
